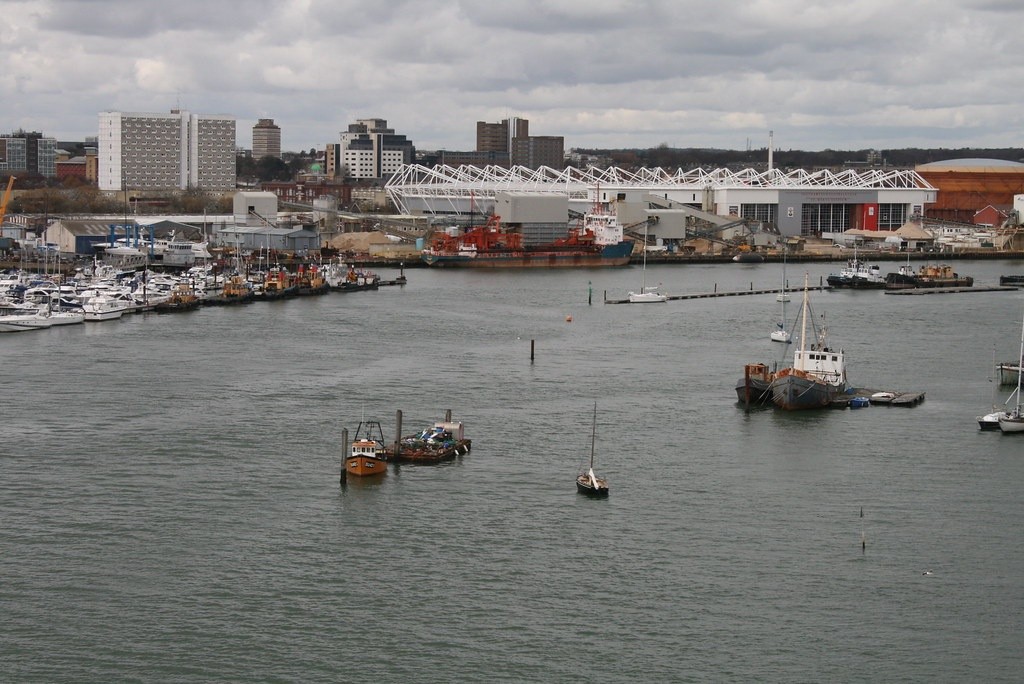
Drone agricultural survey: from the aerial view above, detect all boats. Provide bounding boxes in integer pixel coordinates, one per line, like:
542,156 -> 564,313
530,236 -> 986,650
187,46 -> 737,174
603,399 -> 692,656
385,407 -> 471,462
994,353 -> 1024,386
346,403 -> 389,477
734,362 -> 776,406
824,241 -> 886,288
884,249 -> 974,291
419,183 -> 636,268
978,410 -> 1007,429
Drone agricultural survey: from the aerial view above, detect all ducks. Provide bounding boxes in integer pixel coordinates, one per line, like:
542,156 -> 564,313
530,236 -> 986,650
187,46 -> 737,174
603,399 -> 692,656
923,569 -> 933,575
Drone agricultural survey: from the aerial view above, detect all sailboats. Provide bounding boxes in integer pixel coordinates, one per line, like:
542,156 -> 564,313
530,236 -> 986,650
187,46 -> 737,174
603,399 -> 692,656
776,251 -> 791,301
771,290 -> 790,343
772,269 -> 850,410
0,213 -> 380,335
575,399 -> 610,494
626,224 -> 668,303
997,315 -> 1024,434
